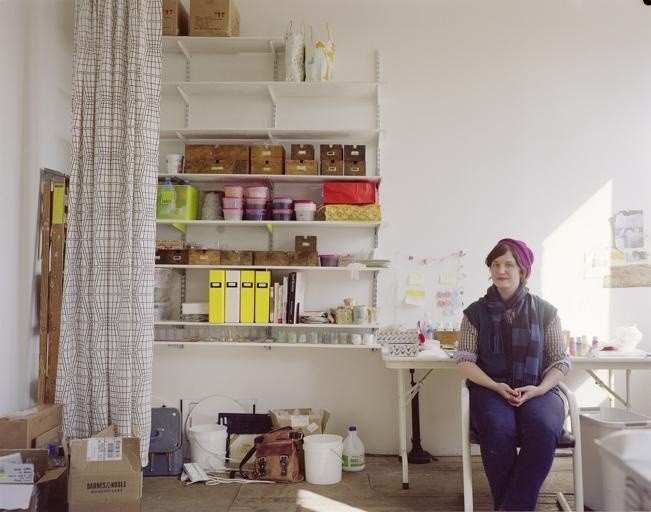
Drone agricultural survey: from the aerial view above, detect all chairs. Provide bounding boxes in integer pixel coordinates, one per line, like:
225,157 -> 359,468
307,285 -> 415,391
461,382 -> 586,511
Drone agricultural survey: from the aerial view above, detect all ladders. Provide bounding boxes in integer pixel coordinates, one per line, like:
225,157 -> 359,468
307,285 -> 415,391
35,167 -> 69,403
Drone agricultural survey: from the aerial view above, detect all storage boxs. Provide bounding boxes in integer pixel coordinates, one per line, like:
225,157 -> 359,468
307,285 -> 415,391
268,408 -> 331,437
156,144 -> 381,267
1,403 -> 142,511
161,1 -> 240,37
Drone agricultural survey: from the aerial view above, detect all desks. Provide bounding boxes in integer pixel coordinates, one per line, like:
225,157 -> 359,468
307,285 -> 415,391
382,344 -> 650,488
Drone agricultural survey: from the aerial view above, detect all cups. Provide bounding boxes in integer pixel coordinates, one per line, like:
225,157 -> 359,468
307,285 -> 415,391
165,154 -> 184,175
154,327 -> 377,346
616,327 -> 643,353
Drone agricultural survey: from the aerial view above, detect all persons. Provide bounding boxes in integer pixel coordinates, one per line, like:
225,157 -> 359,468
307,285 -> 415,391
451,237 -> 571,511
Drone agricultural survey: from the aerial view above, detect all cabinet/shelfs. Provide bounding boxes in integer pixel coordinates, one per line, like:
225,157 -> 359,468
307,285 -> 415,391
156,36 -> 383,347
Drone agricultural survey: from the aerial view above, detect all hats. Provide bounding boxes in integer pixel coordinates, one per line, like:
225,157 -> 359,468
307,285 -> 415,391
498,239 -> 534,279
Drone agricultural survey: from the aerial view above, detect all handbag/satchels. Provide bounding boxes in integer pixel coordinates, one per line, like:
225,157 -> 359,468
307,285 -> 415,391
285,16 -> 304,82
143,406 -> 184,476
316,182 -> 382,221
304,23 -> 336,82
239,426 -> 304,483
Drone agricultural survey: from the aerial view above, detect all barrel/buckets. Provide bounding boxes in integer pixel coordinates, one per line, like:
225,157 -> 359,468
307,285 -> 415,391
187,424 -> 229,471
301,433 -> 344,484
342,427 -> 365,472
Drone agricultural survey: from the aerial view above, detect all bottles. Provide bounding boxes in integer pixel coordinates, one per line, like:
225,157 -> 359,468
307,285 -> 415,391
570,335 -> 601,355
420,311 -> 434,341
353,304 -> 368,324
156,177 -> 177,219
